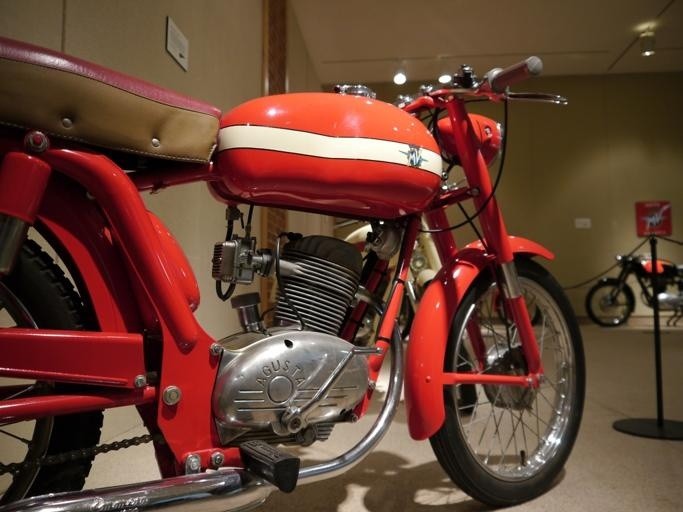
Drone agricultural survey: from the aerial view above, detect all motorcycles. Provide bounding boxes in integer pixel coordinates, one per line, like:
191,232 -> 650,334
586,253 -> 683,327
0,36 -> 586,512
